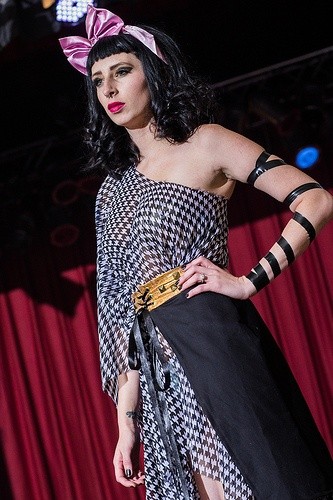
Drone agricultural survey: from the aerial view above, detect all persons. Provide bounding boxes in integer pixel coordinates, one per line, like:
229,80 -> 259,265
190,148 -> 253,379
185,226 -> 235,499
74,19 -> 333,499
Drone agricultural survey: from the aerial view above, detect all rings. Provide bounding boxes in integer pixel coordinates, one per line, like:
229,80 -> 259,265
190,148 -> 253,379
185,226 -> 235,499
199,273 -> 206,284
